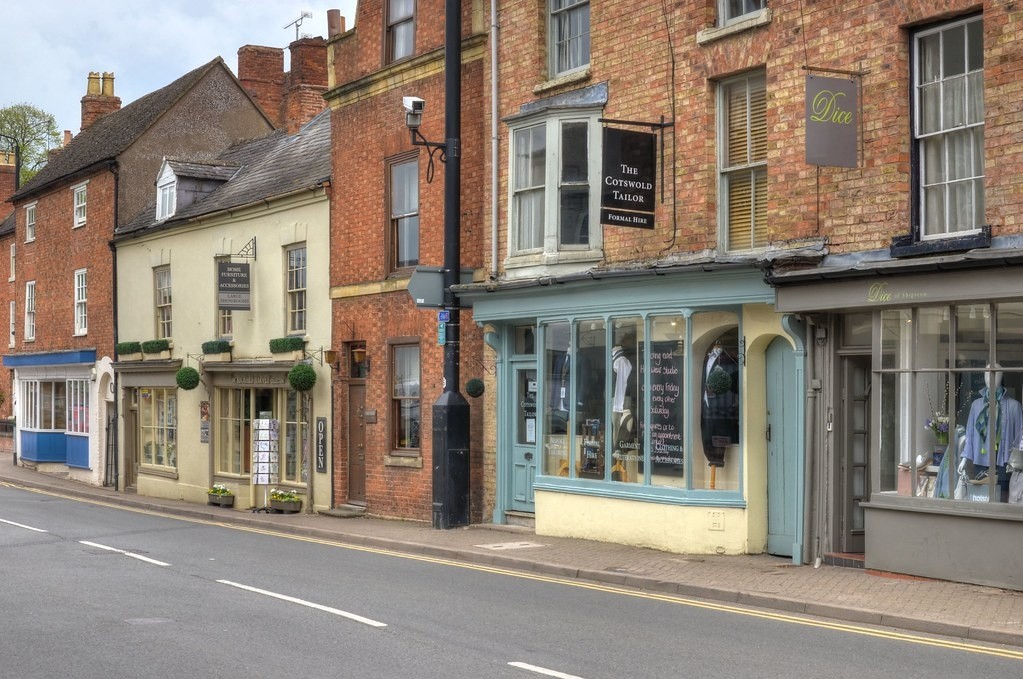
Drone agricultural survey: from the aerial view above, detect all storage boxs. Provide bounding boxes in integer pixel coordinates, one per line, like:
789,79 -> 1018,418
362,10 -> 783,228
967,484 -> 1001,502
898,470 -> 925,496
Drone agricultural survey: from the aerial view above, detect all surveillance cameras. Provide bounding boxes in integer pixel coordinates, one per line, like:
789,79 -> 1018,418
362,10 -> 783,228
403,97 -> 425,115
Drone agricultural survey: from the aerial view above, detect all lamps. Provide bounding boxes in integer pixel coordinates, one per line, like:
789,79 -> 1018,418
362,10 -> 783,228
982,306 -> 990,318
943,309 -> 949,320
969,307 -> 977,319
904,313 -> 911,322
323,349 -> 341,372
352,348 -> 371,372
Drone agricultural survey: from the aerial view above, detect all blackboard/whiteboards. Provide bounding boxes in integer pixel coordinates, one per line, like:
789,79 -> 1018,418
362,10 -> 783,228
637,339 -> 683,477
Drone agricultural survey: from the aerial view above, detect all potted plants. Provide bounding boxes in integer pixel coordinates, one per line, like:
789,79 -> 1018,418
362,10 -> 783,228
201,340 -> 233,362
140,339 -> 171,361
115,342 -> 143,362
268,338 -> 305,362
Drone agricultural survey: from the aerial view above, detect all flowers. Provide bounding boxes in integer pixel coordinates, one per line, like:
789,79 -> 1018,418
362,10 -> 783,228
271,488 -> 301,502
924,380 -> 973,435
208,483 -> 233,496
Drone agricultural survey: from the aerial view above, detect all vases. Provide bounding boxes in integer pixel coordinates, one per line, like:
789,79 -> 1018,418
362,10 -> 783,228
269,500 -> 302,513
936,432 -> 949,446
208,494 -> 233,507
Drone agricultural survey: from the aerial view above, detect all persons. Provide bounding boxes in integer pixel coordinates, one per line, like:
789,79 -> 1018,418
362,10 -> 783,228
701,346 -> 735,466
957,363 -> 1023,503
550,346 -> 589,434
609,346 -> 636,454
